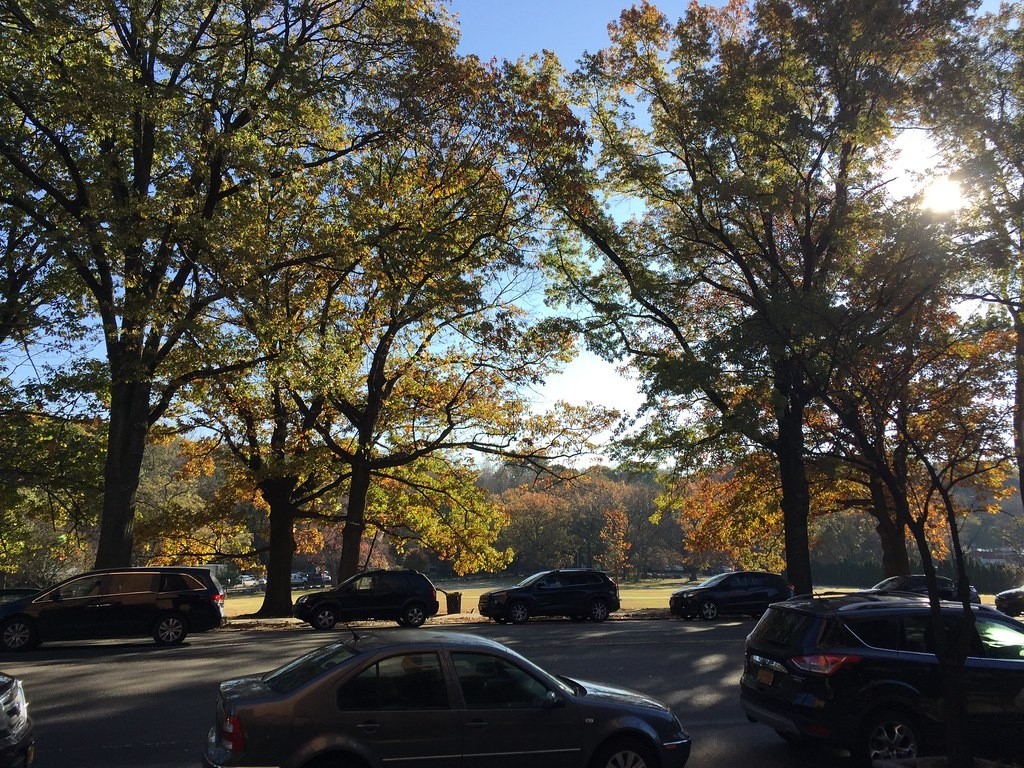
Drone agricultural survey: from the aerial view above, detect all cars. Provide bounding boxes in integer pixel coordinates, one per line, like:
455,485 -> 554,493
995,583 -> 1024,615
872,574 -> 982,604
199,628 -> 695,768
225,571 -> 332,589
0,670 -> 38,768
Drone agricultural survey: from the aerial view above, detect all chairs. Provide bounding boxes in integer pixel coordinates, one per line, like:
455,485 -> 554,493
399,657 -> 447,710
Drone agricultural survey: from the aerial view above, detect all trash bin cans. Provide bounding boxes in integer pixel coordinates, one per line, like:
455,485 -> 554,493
446,591 -> 462,614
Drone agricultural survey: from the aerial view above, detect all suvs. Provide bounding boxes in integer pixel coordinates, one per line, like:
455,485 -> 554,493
741,590 -> 1024,762
293,569 -> 438,629
669,572 -> 796,621
477,569 -> 621,623
0,566 -> 228,657
